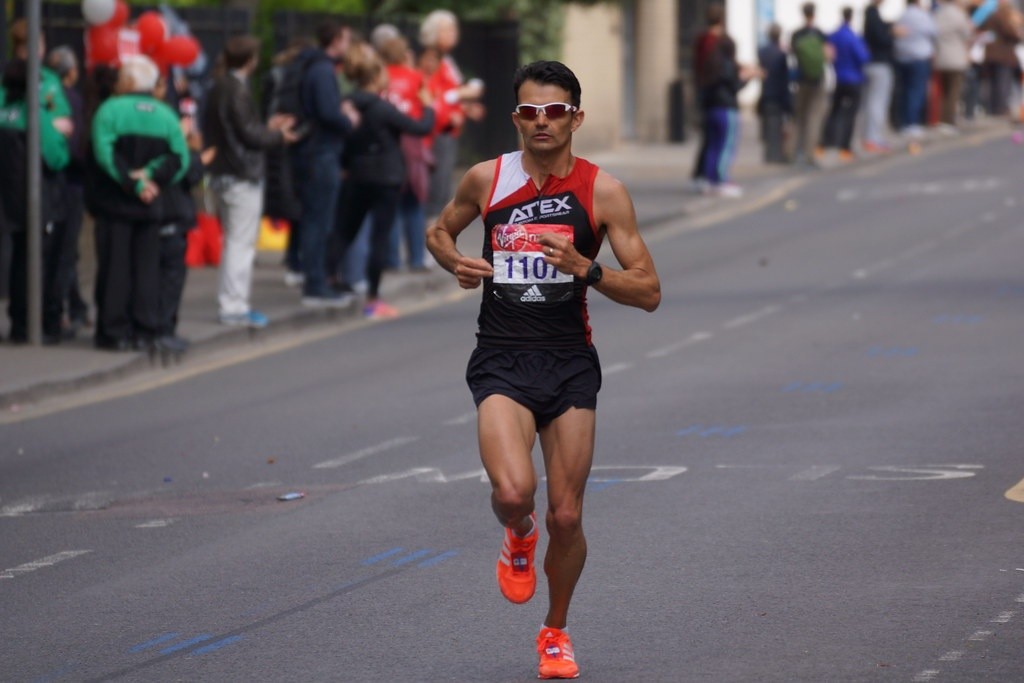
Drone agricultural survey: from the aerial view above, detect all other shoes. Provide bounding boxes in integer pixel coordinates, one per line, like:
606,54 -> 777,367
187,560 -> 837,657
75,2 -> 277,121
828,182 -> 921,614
285,273 -> 305,286
156,335 -> 185,354
221,312 -> 270,328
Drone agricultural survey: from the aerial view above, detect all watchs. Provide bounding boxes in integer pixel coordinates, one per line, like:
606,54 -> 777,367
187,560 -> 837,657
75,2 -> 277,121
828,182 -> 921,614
581,261 -> 603,287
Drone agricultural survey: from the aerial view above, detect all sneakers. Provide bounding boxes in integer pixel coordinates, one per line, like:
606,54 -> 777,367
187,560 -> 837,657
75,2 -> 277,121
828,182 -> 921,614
496,510 -> 538,604
305,292 -> 354,309
536,623 -> 580,679
364,298 -> 399,318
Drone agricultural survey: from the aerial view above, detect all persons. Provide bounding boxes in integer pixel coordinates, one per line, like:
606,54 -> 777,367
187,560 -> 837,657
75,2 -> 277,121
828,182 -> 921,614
425,59 -> 662,680
757,0 -> 1024,165
683,6 -> 769,200
0,9 -> 486,353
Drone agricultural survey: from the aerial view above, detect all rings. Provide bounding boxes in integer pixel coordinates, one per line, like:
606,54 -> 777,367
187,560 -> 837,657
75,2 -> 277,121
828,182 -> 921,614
548,247 -> 554,256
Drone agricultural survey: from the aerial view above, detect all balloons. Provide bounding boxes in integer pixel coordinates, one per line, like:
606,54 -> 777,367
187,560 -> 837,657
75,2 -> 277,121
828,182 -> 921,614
81,0 -> 199,66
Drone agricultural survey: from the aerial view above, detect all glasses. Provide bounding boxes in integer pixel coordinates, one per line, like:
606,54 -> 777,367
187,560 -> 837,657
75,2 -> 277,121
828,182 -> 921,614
516,103 -> 577,120
